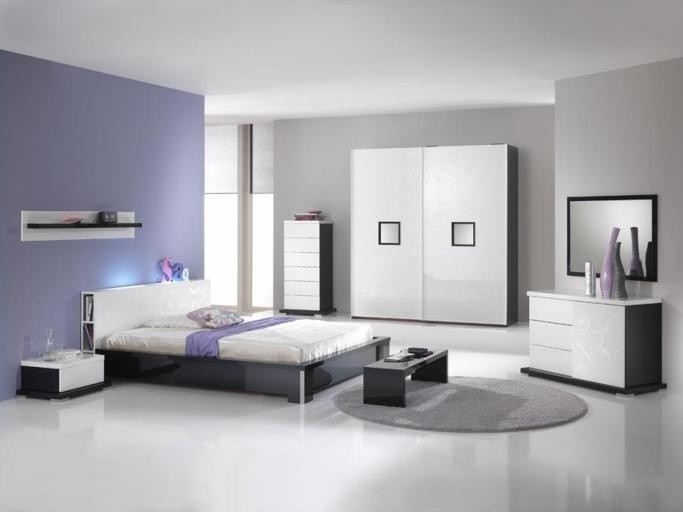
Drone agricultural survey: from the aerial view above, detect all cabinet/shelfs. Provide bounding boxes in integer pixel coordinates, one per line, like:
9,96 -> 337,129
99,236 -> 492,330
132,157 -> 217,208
279,220 -> 337,316
350,144 -> 518,327
521,290 -> 667,398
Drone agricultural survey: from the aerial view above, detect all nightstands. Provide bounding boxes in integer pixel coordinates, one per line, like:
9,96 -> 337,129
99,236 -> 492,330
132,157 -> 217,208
15,354 -> 112,402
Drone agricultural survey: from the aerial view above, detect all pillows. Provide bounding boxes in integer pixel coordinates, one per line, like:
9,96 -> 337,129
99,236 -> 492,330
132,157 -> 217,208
143,315 -> 202,329
185,306 -> 245,329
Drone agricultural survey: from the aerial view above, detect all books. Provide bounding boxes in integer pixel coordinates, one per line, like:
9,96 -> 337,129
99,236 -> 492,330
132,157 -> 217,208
383,347 -> 433,362
293,210 -> 327,220
82,324 -> 92,351
58,216 -> 81,224
84,296 -> 92,321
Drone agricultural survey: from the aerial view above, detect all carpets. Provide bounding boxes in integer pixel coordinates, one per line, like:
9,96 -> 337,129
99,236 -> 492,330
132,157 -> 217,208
333,376 -> 587,433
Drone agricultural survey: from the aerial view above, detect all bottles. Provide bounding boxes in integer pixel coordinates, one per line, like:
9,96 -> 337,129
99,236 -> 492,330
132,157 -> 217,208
42,327 -> 56,362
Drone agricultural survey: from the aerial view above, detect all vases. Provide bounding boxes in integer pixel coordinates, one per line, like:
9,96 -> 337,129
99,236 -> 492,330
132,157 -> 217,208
600,227 -> 629,298
630,227 -> 653,278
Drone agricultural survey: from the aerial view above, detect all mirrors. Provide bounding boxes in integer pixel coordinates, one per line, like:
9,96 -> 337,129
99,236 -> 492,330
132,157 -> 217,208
566,194 -> 658,282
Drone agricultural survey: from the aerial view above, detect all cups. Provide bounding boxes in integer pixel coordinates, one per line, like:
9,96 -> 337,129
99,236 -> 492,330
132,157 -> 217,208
55,338 -> 63,361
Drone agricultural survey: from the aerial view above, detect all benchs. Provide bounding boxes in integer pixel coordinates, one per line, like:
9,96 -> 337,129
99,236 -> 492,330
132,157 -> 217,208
363,347 -> 449,408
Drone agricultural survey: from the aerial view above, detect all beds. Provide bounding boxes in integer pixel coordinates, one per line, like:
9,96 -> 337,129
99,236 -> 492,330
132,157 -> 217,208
80,279 -> 390,405
